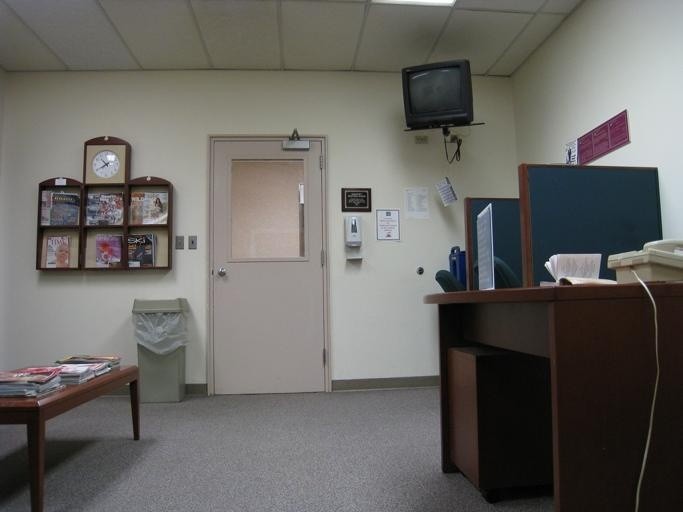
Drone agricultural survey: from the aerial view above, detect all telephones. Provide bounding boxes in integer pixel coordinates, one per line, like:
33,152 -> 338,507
607,240 -> 682,284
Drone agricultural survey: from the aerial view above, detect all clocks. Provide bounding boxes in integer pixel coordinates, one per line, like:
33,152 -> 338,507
83,134 -> 131,185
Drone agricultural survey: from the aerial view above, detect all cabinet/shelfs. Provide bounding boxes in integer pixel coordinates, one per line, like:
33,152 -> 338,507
34,176 -> 173,270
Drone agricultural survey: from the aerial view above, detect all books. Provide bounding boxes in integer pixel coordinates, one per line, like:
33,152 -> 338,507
538,252 -> 617,287
41,191 -> 169,268
0,352 -> 121,397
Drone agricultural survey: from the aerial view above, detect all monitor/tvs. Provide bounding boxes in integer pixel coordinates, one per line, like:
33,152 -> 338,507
401,57 -> 473,128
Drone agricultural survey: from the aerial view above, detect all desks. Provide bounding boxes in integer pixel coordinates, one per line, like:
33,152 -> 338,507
0,365 -> 142,511
419,281 -> 678,512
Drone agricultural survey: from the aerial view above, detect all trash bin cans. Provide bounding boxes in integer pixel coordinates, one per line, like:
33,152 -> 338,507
132,298 -> 187,403
